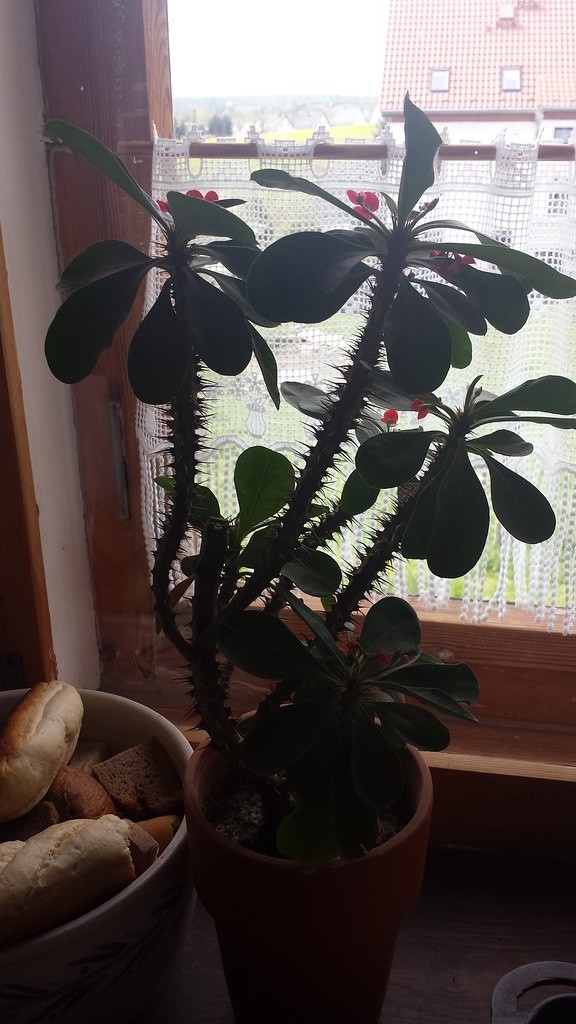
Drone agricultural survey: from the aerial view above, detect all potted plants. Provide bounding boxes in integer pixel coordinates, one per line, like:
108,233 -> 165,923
42,94 -> 576,1023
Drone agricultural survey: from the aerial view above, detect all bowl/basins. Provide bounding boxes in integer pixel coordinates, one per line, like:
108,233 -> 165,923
0,689 -> 194,1024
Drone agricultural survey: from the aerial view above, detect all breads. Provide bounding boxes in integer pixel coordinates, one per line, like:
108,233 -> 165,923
0,679 -> 181,948
